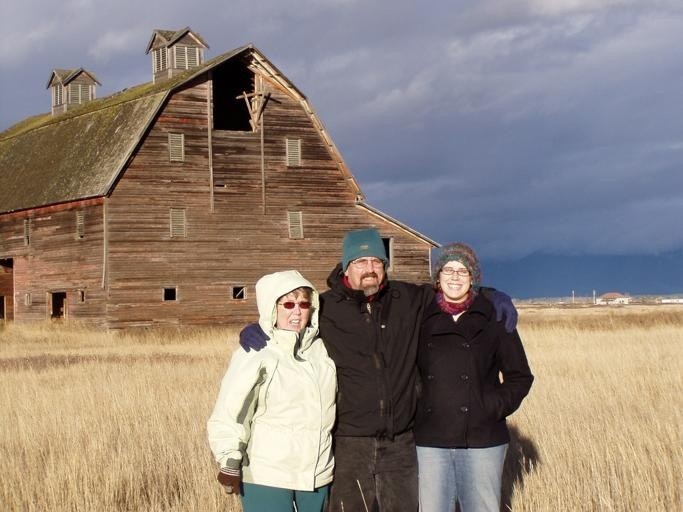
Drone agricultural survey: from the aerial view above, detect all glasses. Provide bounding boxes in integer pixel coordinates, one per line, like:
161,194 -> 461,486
352,259 -> 383,269
279,301 -> 310,310
441,266 -> 470,276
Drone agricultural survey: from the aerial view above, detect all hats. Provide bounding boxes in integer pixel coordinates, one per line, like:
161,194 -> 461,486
341,228 -> 390,273
431,241 -> 480,293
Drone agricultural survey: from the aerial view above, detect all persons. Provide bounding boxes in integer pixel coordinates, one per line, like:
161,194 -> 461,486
414,241 -> 534,512
207,270 -> 336,512
239,229 -> 518,511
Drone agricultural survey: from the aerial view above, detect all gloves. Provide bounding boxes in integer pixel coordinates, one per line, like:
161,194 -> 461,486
493,290 -> 519,332
215,467 -> 242,497
238,322 -> 269,352
